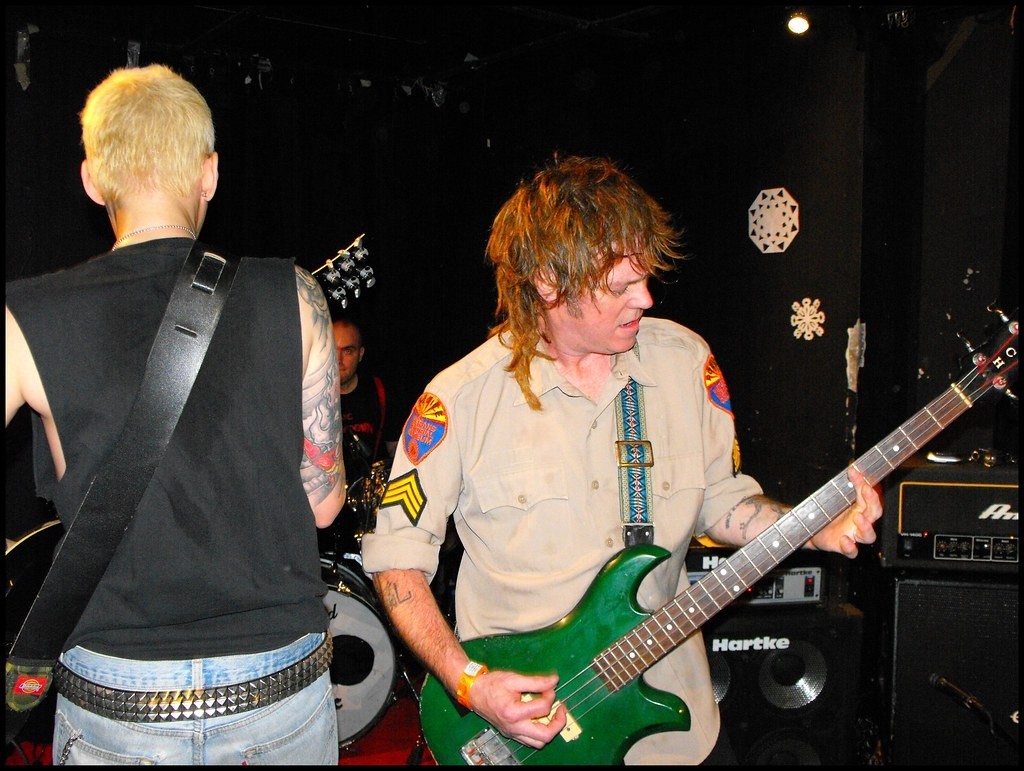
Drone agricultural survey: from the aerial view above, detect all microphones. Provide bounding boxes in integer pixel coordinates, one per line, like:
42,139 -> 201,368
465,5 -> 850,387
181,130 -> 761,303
928,672 -> 984,711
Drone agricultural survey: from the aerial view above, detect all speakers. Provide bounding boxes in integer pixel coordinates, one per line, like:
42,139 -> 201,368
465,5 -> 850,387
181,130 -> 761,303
696,600 -> 867,768
860,565 -> 1023,768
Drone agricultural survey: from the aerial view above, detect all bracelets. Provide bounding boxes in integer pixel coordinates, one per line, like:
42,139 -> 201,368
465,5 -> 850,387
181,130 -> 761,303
456,661 -> 489,712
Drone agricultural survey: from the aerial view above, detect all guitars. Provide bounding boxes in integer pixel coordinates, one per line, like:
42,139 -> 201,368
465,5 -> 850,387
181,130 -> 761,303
1,229 -> 379,744
416,269 -> 1022,767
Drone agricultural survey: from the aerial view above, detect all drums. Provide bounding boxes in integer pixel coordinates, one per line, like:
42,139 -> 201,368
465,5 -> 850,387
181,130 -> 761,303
318,551 -> 404,751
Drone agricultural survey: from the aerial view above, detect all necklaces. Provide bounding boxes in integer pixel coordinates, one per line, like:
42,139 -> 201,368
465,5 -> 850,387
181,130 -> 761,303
112,225 -> 196,251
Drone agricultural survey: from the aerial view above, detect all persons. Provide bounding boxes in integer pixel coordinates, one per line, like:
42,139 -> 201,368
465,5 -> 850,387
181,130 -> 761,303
5,64 -> 346,765
326,309 -> 458,599
362,155 -> 884,765
